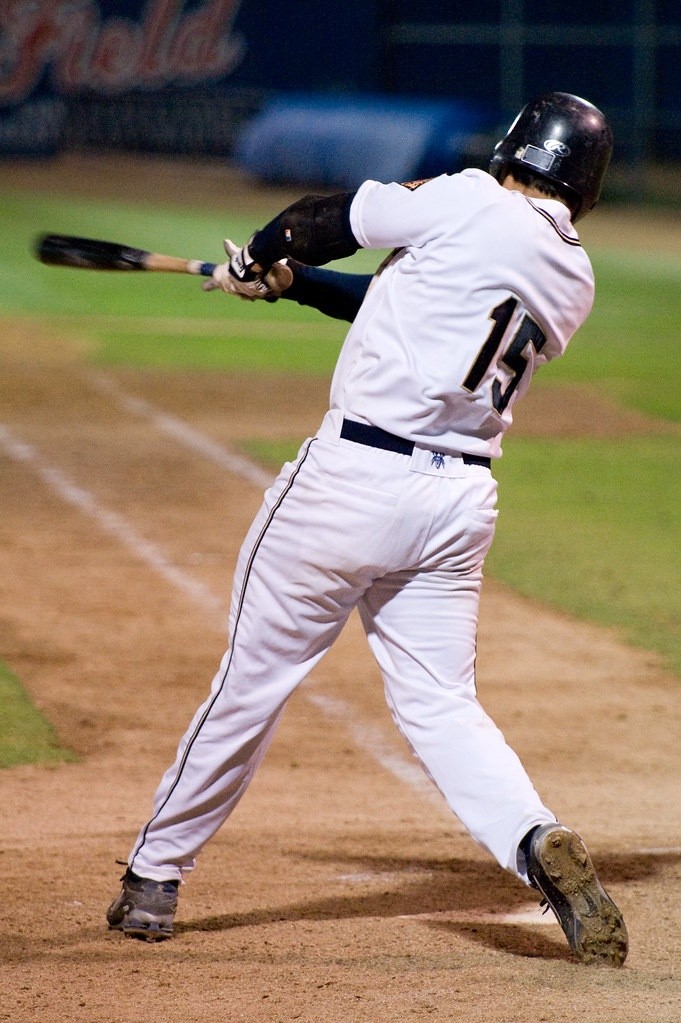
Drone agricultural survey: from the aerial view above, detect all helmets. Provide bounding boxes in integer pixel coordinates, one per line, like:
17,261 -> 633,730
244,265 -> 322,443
488,92 -> 612,225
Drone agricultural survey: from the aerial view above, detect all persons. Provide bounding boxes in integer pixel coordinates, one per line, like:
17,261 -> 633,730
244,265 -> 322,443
106,93 -> 628,966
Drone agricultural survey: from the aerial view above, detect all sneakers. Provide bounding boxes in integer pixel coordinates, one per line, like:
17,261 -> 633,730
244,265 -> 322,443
107,860 -> 180,943
526,823 -> 628,968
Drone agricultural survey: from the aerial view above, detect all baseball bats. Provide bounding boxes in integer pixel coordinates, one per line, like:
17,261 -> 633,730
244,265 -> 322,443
29,233 -> 294,294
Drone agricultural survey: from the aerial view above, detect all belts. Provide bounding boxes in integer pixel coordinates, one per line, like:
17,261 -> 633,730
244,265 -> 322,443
341,418 -> 491,469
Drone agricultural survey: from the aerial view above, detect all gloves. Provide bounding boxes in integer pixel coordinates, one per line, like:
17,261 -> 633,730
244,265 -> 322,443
201,230 -> 294,303
